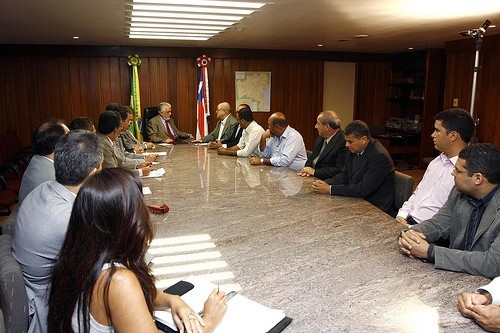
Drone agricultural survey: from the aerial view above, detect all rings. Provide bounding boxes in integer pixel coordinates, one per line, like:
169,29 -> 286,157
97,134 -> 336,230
188,312 -> 196,320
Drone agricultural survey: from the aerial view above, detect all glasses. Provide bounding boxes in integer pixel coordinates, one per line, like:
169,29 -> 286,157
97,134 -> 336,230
126,120 -> 133,125
453,166 -> 484,175
216,109 -> 222,111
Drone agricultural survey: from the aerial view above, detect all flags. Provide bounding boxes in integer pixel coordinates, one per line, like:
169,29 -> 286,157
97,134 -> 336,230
128,66 -> 145,143
196,67 -> 211,141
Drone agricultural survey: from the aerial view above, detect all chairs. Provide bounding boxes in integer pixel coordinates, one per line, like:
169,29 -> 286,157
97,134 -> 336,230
394,171 -> 415,210
144,107 -> 159,131
0,130 -> 34,333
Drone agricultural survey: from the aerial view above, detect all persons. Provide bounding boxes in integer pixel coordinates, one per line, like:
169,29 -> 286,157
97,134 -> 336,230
397,145 -> 500,333
208,104 -> 251,149
147,102 -> 195,144
19,118 -> 71,206
250,112 -> 308,171
42,169 -> 228,333
217,108 -> 266,158
313,121 -> 395,218
235,157 -> 303,207
298,105 -> 347,180
191,102 -> 238,143
97,103 -> 159,176
68,117 -> 97,135
11,131 -> 106,333
395,108 -> 475,239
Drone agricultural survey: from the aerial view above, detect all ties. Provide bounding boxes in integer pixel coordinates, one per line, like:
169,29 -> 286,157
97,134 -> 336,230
165,120 -> 176,145
312,138 -> 327,168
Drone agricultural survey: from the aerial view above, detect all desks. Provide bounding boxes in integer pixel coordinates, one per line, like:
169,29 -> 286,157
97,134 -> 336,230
140,143 -> 500,333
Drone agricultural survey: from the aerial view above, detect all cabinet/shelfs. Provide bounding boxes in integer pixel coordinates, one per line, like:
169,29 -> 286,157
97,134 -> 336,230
387,48 -> 447,170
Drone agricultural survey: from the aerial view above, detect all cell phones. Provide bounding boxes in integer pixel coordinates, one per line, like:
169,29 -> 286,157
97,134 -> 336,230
163,281 -> 194,296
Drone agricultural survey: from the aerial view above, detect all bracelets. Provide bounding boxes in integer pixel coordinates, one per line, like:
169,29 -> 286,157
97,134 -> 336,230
260,168 -> 263,171
260,158 -> 263,164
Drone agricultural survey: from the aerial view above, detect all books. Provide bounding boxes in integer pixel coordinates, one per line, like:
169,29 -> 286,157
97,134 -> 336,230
153,275 -> 293,333
